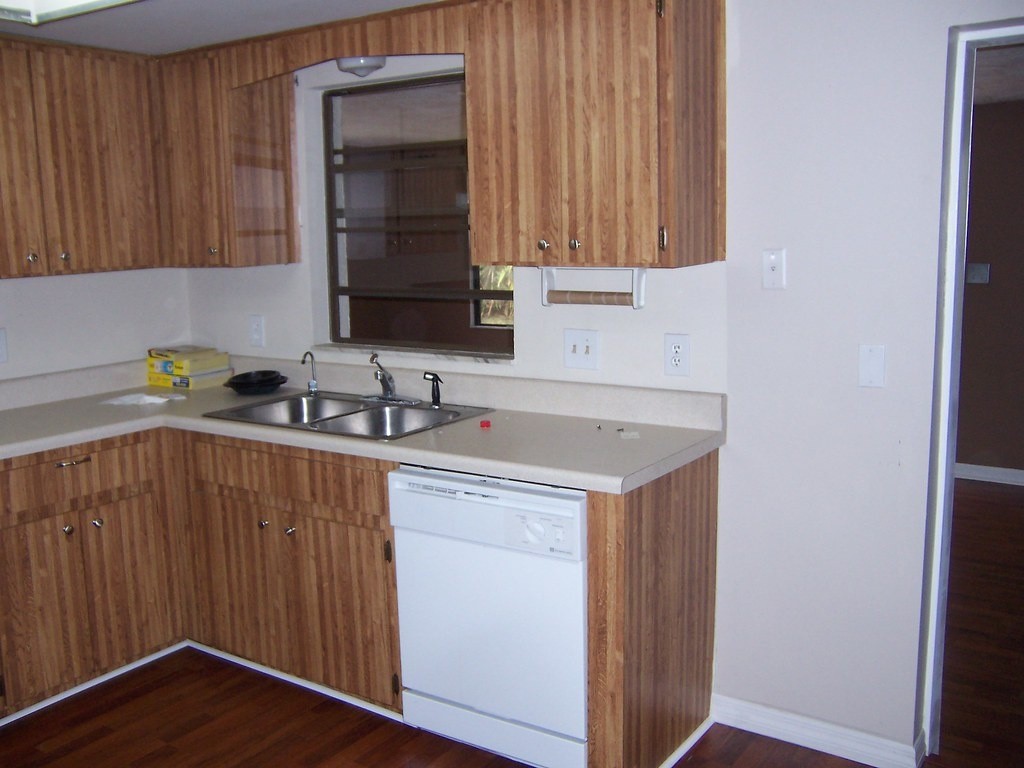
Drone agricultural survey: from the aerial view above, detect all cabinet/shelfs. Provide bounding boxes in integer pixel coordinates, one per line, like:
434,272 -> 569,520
0,427 -> 188,726
156,50 -> 300,269
0,31 -> 161,279
464,0 -> 726,268
164,427 -> 404,716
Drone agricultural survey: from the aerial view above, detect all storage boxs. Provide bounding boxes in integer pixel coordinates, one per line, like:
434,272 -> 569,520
146,352 -> 230,377
146,367 -> 234,390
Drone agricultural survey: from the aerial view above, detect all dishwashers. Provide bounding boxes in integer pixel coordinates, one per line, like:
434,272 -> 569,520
387,463 -> 588,768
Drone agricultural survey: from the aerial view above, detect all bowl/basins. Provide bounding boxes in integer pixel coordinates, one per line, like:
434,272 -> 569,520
222,370 -> 287,394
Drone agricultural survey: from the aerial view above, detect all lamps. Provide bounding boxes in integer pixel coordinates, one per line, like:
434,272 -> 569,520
337,57 -> 385,77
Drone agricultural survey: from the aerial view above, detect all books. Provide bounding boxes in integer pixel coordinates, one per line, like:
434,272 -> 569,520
147,344 -> 234,390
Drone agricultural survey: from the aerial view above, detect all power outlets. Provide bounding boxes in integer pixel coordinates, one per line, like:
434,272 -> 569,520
248,313 -> 266,346
663,333 -> 691,378
761,246 -> 786,291
564,327 -> 602,370
